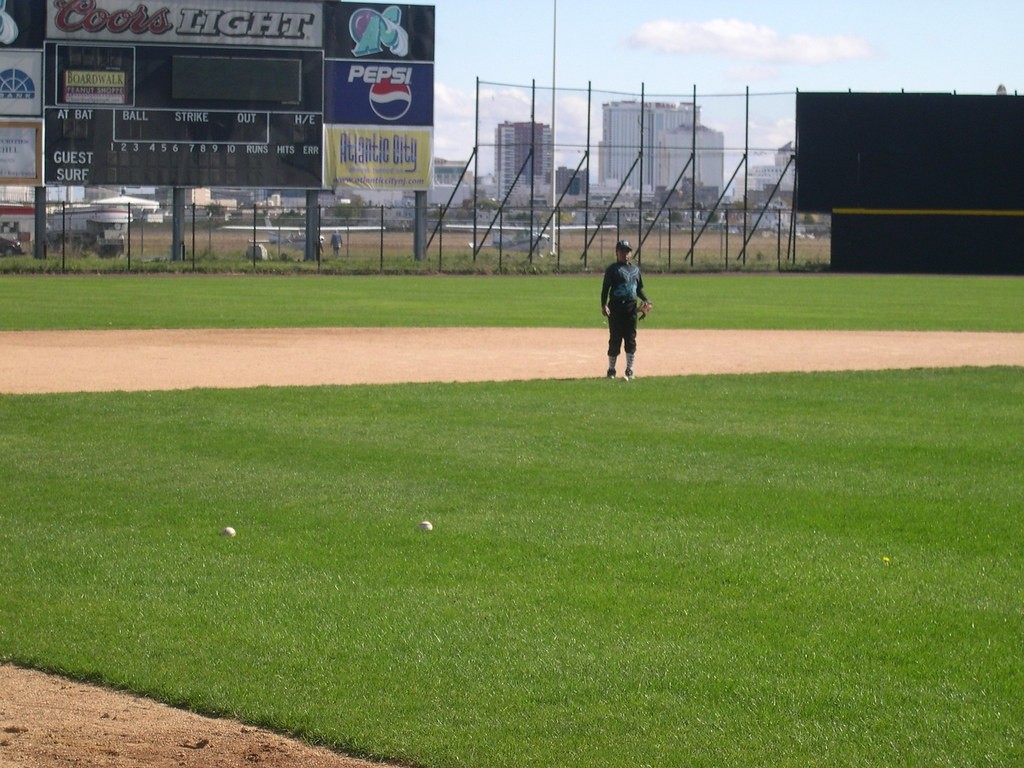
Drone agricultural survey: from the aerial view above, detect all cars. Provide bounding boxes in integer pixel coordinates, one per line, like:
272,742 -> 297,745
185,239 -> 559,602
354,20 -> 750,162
0,238 -> 21,258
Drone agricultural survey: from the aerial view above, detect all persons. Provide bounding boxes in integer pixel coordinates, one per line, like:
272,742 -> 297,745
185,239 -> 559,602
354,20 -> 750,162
320,230 -> 325,255
330,230 -> 343,255
600,239 -> 653,379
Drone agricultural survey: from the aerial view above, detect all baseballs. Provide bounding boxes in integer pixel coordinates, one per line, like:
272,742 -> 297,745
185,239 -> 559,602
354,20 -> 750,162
419,521 -> 433,531
222,526 -> 236,536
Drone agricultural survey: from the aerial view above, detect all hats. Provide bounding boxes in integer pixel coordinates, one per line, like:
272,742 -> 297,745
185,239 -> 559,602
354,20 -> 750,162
617,240 -> 633,251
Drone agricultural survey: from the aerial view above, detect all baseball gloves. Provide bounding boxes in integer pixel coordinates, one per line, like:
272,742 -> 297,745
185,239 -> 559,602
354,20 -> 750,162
633,301 -> 652,321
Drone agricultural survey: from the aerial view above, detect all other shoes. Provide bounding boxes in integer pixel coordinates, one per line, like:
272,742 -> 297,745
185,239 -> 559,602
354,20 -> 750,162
624,368 -> 633,379
607,370 -> 617,379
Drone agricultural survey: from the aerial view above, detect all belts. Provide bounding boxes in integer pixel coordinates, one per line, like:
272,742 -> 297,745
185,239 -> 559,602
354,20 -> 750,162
612,300 -> 636,304
